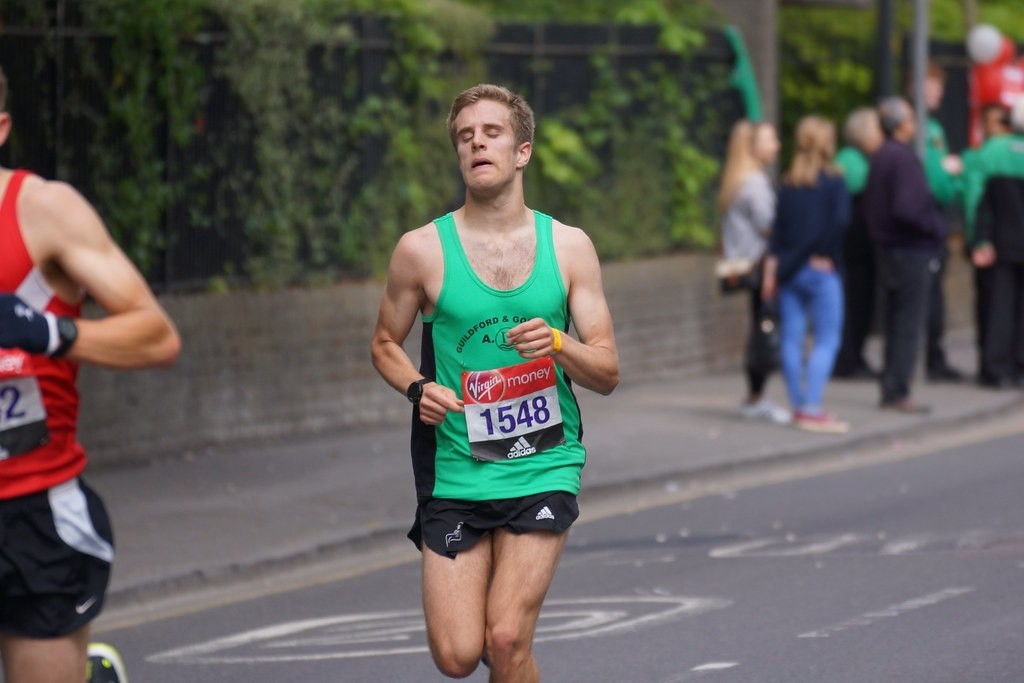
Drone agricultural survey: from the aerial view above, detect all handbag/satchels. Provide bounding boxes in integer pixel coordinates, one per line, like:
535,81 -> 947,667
716,212 -> 764,294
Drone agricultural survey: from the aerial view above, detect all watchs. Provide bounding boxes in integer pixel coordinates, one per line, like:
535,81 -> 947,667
48,315 -> 78,358
406,377 -> 435,407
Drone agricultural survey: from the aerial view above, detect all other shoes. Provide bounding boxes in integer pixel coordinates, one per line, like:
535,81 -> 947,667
925,363 -> 972,381
737,400 -> 790,422
877,396 -> 933,413
977,372 -> 1023,390
833,359 -> 884,379
794,412 -> 848,432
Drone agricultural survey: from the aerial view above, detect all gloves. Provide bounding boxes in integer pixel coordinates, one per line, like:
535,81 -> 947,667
0,293 -> 60,356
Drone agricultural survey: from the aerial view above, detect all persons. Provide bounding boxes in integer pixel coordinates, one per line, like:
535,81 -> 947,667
370,84 -> 621,683
713,59 -> 1024,435
1,74 -> 184,683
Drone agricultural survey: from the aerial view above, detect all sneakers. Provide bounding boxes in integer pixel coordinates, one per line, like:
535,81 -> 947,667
87,642 -> 128,683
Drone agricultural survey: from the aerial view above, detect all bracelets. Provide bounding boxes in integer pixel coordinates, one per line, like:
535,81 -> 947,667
551,328 -> 563,355
